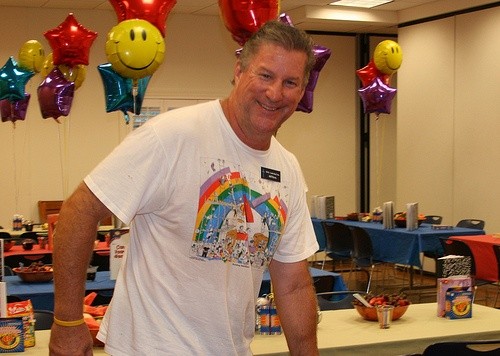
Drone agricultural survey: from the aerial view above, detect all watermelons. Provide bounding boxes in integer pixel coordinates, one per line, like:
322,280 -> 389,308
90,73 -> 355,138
82,291 -> 109,329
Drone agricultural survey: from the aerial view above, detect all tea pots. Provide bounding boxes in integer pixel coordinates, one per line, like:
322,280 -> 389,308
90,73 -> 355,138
86,265 -> 99,280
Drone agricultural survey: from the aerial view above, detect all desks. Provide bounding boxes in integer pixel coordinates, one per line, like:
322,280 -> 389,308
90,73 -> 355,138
0,223 -> 114,240
446,233 -> 500,284
0,237 -> 112,271
311,217 -> 486,293
0,300 -> 500,356
258,265 -> 348,302
0,270 -> 116,314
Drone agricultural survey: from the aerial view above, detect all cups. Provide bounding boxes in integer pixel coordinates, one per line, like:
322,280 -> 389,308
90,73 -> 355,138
38,237 -> 45,249
407,203 -> 418,230
383,201 -> 393,229
23,239 -> 33,251
376,305 -> 394,329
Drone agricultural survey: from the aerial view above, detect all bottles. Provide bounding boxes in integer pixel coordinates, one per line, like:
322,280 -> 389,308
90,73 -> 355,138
13,215 -> 22,229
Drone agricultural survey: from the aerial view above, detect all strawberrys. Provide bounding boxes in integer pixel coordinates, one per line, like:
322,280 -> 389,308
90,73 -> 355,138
367,293 -> 410,307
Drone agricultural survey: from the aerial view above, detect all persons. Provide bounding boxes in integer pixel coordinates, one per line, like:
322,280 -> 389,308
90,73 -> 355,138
49,24 -> 321,356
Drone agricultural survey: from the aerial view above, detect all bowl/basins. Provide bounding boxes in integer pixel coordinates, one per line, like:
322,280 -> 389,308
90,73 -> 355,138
354,304 -> 410,321
394,220 -> 424,227
0,241 -> 17,251
12,267 -> 53,281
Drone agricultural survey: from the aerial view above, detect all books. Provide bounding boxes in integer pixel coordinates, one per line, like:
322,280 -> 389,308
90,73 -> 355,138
383,201 -> 395,230
405,202 -> 419,231
436,254 -> 471,316
309,194 -> 335,220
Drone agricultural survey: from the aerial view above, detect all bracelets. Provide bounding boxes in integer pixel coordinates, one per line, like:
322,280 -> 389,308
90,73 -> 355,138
54,317 -> 85,329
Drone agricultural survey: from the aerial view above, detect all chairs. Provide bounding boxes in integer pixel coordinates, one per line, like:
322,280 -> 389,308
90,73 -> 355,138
31,309 -> 55,331
348,225 -> 394,297
438,235 -> 495,305
422,339 -> 500,356
38,200 -> 64,224
319,222 -> 356,287
423,215 -> 442,224
492,244 -> 500,310
457,219 -> 485,230
0,229 -> 109,306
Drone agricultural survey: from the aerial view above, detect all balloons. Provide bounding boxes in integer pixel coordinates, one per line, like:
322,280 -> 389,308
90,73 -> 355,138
356,40 -> 404,114
97,0 -> 176,126
219,0 -> 331,114
1,13 -> 99,126
